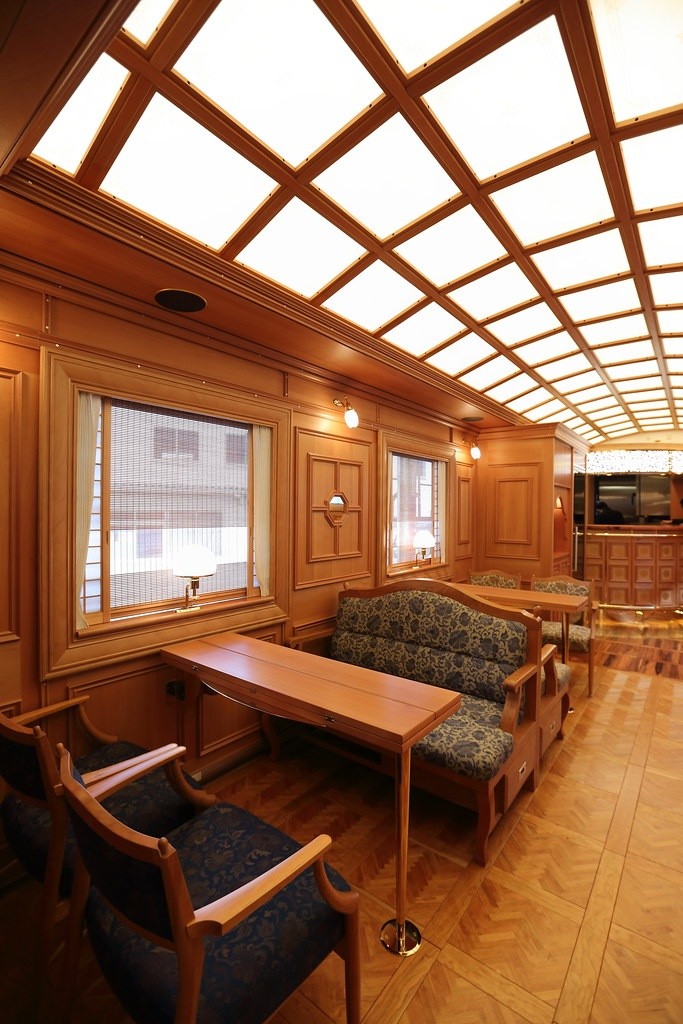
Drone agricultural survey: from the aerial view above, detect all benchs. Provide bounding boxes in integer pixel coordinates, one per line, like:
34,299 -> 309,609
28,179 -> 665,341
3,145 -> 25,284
260,577 -> 540,869
343,577 -> 571,787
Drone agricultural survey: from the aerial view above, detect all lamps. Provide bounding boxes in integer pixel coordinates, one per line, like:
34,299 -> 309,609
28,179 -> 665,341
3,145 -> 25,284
172,544 -> 218,612
462,439 -> 482,460
333,396 -> 359,428
412,530 -> 436,569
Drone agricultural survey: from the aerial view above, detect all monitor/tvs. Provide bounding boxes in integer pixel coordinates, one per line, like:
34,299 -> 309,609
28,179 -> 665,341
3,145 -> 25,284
593,472 -> 672,526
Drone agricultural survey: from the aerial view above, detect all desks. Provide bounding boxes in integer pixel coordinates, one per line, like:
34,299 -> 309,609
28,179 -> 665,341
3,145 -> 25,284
159,632 -> 461,958
451,583 -> 589,714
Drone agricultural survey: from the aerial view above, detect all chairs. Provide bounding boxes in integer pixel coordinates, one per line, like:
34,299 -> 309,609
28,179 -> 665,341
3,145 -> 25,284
467,568 -> 521,590
49,742 -> 361,1024
0,695 -> 218,1018
528,575 -> 595,698
